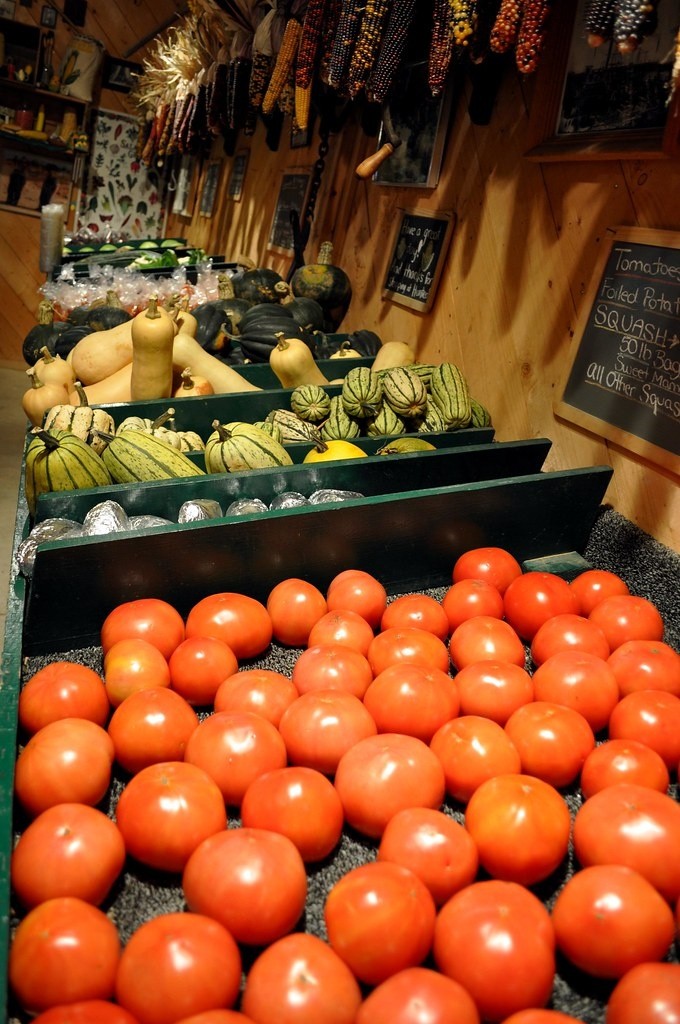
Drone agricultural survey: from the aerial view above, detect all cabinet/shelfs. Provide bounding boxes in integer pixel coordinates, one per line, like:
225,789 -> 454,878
0,16 -> 89,152
0,238 -> 679,1024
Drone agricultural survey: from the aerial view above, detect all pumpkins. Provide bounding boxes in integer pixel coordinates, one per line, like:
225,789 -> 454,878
22,242 -> 491,527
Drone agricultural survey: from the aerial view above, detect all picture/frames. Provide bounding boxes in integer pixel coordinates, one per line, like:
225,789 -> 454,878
199,158 -> 222,217
554,224 -> 680,480
170,154 -> 200,217
381,207 -> 456,312
371,59 -> 456,189
0,149 -> 74,222
266,165 -> 314,258
226,149 -> 250,201
556,0 -> 680,138
291,114 -> 312,147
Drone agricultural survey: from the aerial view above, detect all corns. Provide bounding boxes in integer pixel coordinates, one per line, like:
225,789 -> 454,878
135,0 -> 660,168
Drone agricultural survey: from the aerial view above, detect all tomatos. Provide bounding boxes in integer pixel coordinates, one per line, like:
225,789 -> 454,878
10,547 -> 680,1024
